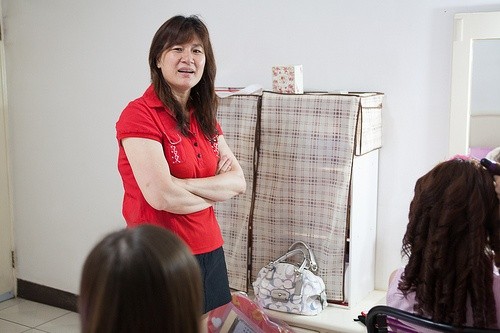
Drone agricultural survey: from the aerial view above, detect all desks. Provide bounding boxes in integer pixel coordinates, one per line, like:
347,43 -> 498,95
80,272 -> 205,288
261,306 -> 387,333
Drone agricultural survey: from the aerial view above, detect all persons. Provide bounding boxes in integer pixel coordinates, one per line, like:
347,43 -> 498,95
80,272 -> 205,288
116,16 -> 247,333
387,159 -> 500,333
80,225 -> 203,333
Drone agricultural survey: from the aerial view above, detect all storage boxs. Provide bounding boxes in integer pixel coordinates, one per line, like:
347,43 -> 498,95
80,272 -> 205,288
272,64 -> 304,95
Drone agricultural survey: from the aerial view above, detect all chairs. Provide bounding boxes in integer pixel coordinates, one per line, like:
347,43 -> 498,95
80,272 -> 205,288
366,305 -> 500,333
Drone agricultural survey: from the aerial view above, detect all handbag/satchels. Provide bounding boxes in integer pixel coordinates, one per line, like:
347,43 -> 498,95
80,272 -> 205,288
253,241 -> 328,315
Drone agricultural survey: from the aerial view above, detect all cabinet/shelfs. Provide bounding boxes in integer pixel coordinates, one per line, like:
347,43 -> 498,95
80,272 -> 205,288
209,88 -> 384,308
